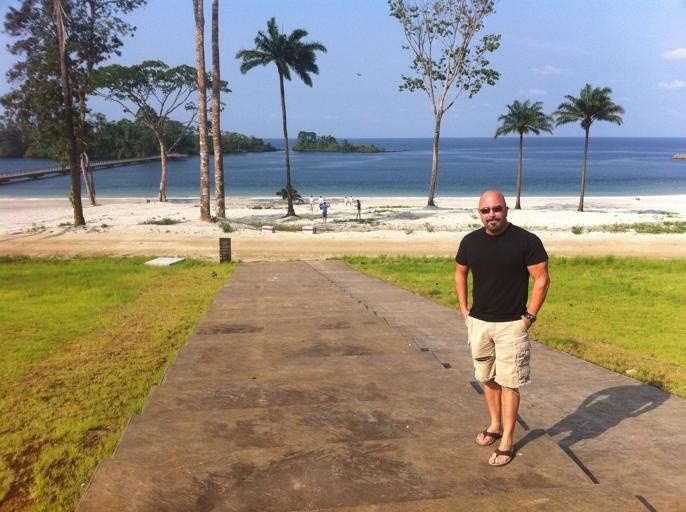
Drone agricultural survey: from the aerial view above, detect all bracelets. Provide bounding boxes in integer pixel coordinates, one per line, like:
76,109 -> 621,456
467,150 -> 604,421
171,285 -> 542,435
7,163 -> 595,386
524,311 -> 536,322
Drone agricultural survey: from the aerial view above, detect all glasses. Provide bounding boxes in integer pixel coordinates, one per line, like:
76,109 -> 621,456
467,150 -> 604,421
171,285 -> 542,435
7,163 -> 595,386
479,206 -> 504,214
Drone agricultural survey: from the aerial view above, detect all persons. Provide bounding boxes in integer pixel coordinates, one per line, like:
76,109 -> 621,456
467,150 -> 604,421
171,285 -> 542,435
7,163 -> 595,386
344,196 -> 348,207
454,190 -> 551,466
318,195 -> 323,208
349,196 -> 352,206
319,202 -> 330,224
354,199 -> 361,219
309,196 -> 314,211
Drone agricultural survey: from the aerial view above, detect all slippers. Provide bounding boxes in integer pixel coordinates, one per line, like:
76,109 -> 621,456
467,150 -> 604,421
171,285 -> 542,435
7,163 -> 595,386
488,447 -> 514,466
475,429 -> 502,447
